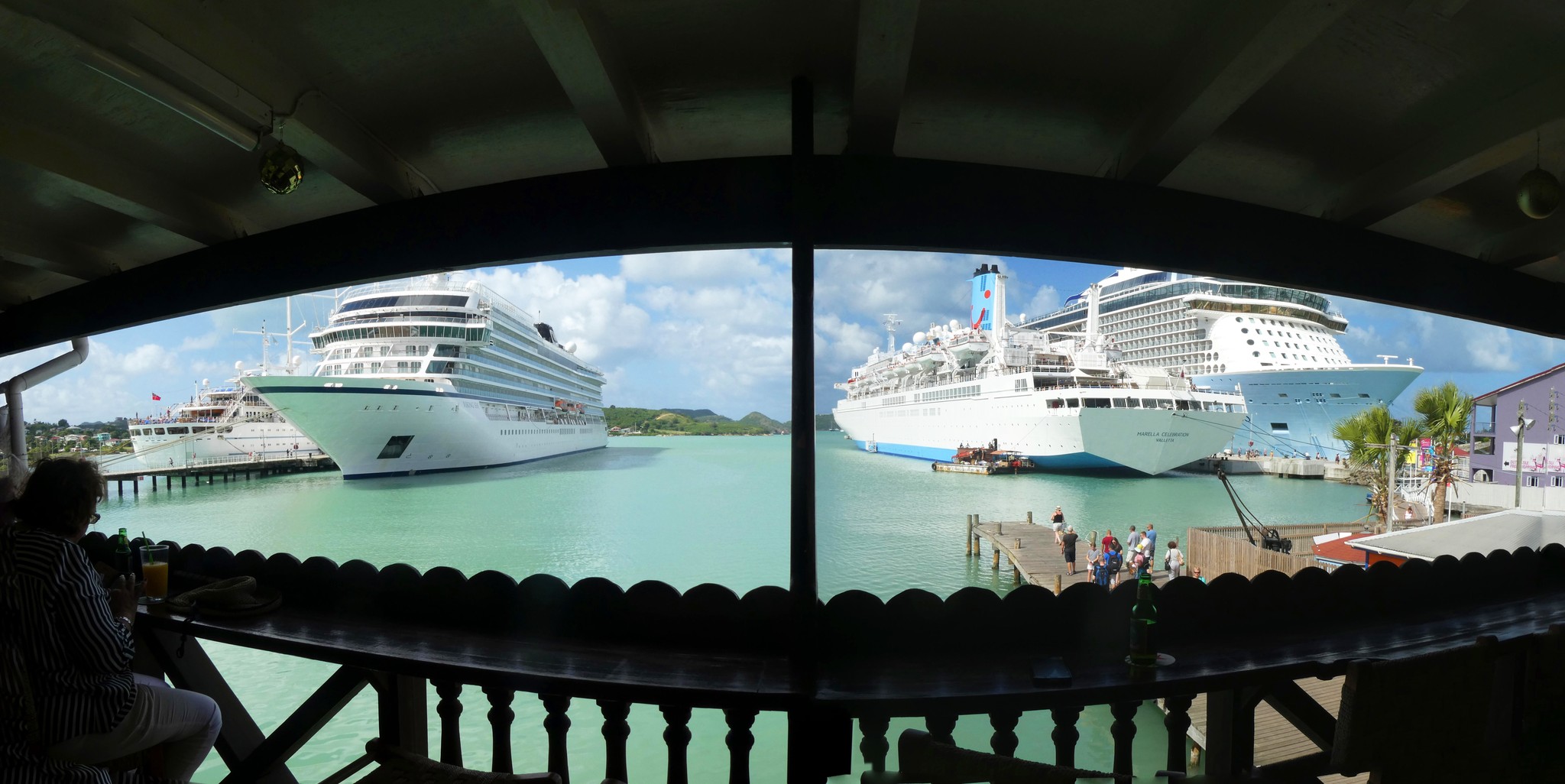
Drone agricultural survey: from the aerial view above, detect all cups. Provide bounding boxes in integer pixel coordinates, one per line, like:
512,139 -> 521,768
139,544 -> 169,601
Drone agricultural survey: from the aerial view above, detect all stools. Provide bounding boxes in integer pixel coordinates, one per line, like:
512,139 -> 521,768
0,755 -> 137,784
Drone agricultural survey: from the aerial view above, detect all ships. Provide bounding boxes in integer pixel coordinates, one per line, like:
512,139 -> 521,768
124,297 -> 327,468
829,272 -> 1248,476
1006,266 -> 1425,462
235,270 -> 611,480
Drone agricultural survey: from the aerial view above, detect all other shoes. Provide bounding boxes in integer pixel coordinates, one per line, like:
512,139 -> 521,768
1125,566 -> 1130,568
1059,542 -> 1062,545
1054,542 -> 1058,543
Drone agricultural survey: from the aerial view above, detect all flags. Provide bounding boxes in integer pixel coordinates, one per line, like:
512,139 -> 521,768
272,336 -> 278,346
152,393 -> 161,401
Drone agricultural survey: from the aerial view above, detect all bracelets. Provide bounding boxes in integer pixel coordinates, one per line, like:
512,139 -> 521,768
115,616 -> 135,633
1061,550 -> 1063,551
1091,561 -> 1094,564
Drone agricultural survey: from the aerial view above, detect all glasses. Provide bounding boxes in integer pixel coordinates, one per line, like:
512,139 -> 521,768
1194,571 -> 1200,574
1147,527 -> 1149,528
83,506 -> 101,525
1129,528 -> 1131,529
1140,535 -> 1142,536
1108,547 -> 1110,549
1112,542 -> 1115,543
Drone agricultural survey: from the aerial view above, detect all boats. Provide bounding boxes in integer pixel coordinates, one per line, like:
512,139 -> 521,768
554,400 -> 568,413
565,405 -> 578,413
847,378 -> 856,389
931,438 -> 1041,475
829,420 -> 845,432
574,403 -> 585,415
857,358 -> 920,386
914,343 -> 949,364
946,332 -> 991,354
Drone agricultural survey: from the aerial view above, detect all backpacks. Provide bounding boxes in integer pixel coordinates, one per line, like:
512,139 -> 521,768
1141,554 -> 1149,569
1245,451 -> 1246,454
1106,552 -> 1121,574
1096,564 -> 1109,586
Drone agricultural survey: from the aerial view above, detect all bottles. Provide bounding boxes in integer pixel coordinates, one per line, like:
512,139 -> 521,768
1130,573 -> 1157,665
115,528 -> 132,580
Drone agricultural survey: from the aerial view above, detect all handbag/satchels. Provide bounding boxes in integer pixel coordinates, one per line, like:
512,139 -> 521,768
1177,552 -> 1184,566
1165,561 -> 1171,571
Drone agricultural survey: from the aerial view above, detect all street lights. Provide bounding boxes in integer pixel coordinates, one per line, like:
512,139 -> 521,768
36,433 -> 93,460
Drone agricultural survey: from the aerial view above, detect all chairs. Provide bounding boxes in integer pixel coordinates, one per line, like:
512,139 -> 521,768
1330,621 -> 1565,783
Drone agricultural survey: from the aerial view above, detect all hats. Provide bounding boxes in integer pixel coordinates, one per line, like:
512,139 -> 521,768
1264,449 -> 1266,450
1135,544 -> 1143,553
1271,450 -> 1273,452
1256,449 -> 1257,450
1055,506 -> 1062,510
1246,449 -> 1248,451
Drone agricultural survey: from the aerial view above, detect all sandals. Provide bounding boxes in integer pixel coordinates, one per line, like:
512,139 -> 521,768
1066,573 -> 1072,575
1073,571 -> 1076,574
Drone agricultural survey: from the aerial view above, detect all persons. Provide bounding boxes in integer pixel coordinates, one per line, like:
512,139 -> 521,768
167,410 -> 170,419
241,386 -> 244,394
257,451 -> 260,461
0,454 -> 222,784
192,452 -> 196,458
249,451 -> 253,462
290,448 -> 293,458
1403,504 -> 1414,522
1535,446 -> 1546,473
242,401 -> 246,405
1050,506 -> 1207,588
169,458 -> 174,468
225,399 -> 229,406
146,415 -> 149,421
136,412 -> 138,419
160,412 -> 162,419
252,451 -> 256,462
287,449 -> 289,458
149,415 -> 152,421
1208,444 -> 1385,470
